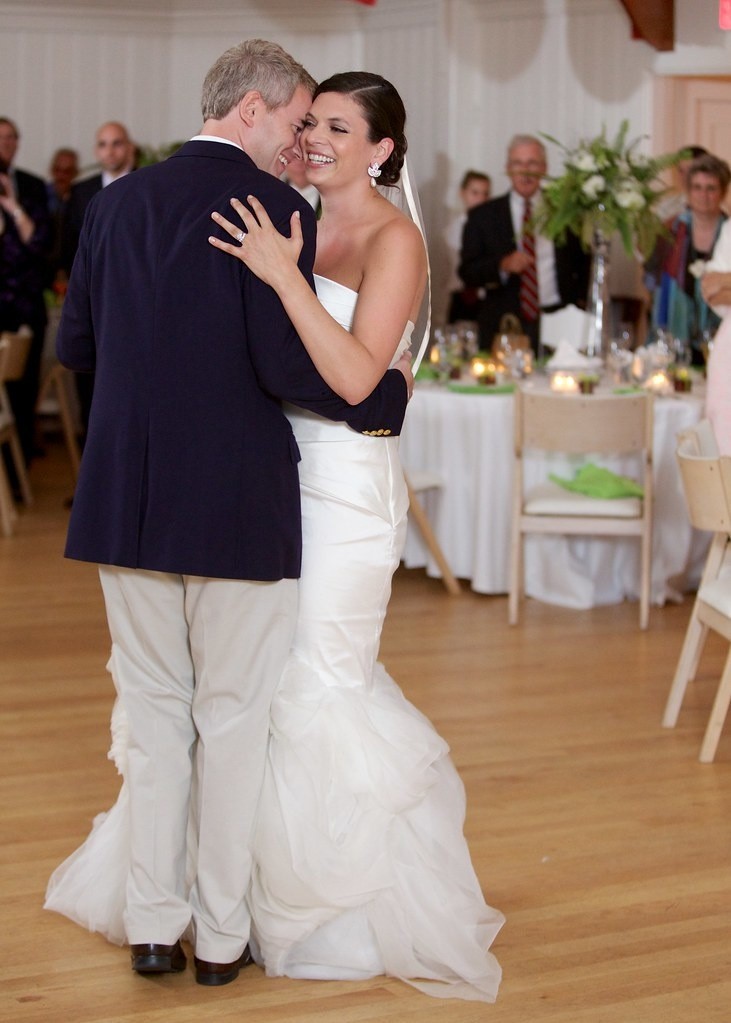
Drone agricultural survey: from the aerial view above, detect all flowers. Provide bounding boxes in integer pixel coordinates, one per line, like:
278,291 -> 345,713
513,117 -> 693,262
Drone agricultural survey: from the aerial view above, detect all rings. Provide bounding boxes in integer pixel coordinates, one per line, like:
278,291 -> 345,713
235,230 -> 246,242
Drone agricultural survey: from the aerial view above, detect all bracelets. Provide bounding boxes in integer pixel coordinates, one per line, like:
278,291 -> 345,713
12,205 -> 25,220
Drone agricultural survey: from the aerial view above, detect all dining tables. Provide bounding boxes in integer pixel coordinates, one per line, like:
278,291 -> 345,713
384,355 -> 702,610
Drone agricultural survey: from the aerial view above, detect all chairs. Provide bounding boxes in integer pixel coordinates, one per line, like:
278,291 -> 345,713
659,418 -> 731,764
0,325 -> 34,539
508,387 -> 658,635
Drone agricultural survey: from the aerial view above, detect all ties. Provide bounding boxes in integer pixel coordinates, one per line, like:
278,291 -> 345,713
521,197 -> 537,323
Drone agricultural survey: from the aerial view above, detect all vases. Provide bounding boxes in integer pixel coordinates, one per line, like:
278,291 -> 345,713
583,231 -> 615,361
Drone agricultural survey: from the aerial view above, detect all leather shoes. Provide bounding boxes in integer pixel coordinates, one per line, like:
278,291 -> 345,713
194,943 -> 252,986
127,943 -> 187,975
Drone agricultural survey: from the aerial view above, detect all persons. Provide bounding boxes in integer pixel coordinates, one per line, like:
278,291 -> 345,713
40,70 -> 508,1004
641,155 -> 731,377
700,206 -> 731,458
55,114 -> 142,509
444,170 -> 492,336
50,36 -> 416,986
672,146 -> 709,185
455,130 -> 588,360
42,146 -> 77,211
0,114 -> 51,462
0,159 -> 46,504
